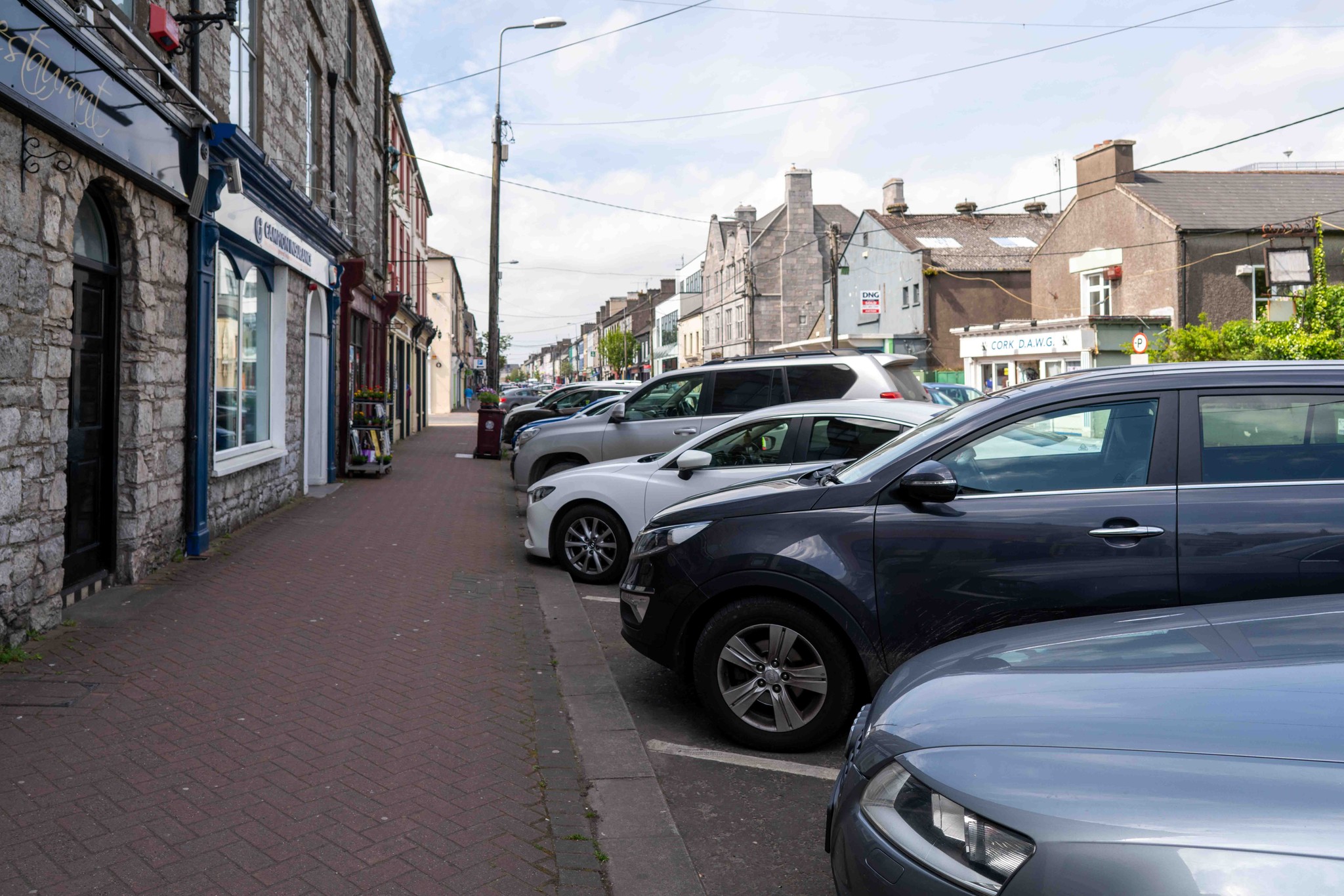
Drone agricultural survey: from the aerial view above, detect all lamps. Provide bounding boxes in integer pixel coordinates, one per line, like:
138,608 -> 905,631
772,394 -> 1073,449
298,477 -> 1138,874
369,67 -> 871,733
264,154 -> 377,246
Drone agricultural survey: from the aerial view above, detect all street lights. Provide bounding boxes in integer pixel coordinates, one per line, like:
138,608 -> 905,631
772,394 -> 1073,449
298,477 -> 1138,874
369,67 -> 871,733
485,15 -> 569,391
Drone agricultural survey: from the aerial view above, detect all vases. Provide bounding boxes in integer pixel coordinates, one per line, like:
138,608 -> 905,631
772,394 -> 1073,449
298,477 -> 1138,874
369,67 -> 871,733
353,422 -> 387,427
378,461 -> 390,465
354,398 -> 385,400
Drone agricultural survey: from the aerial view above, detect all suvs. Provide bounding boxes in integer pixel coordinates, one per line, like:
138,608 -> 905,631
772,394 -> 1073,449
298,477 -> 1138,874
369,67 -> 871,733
503,348 -> 937,493
620,356 -> 1343,754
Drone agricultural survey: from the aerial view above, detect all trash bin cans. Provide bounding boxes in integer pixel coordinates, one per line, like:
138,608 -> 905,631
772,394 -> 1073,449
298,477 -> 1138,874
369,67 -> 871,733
473,408 -> 507,461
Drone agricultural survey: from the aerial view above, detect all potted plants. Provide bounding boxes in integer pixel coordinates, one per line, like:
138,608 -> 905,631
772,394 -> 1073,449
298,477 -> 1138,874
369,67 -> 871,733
349,454 -> 368,465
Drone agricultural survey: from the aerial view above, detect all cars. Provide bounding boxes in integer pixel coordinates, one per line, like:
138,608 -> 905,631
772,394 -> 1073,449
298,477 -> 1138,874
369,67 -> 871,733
923,383 -> 986,406
525,397 -> 1106,586
825,590 -> 1343,896
498,378 -> 563,414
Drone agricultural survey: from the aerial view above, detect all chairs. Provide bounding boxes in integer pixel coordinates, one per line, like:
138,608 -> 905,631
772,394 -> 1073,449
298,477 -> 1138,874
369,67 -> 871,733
819,421 -> 856,461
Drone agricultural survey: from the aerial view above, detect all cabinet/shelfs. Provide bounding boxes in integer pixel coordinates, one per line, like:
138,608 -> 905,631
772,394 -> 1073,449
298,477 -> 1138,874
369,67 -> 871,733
344,398 -> 395,478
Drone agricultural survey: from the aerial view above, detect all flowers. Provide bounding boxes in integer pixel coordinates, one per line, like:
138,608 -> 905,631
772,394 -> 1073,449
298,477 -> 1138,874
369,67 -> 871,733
376,454 -> 392,461
353,410 -> 392,425
356,384 -> 391,399
